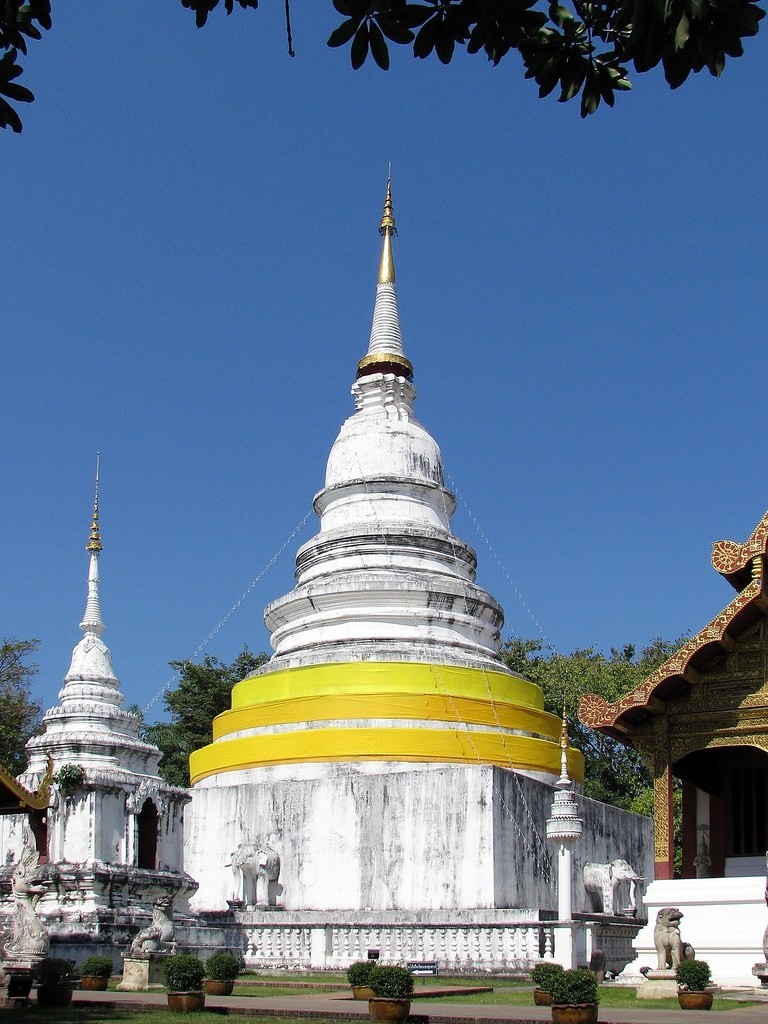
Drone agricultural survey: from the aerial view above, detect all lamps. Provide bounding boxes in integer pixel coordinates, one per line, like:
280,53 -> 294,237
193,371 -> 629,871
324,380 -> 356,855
7,975 -> 33,999
368,949 -> 380,959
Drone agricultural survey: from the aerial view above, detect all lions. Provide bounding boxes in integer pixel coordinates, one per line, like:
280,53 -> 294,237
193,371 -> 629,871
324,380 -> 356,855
653,907 -> 695,970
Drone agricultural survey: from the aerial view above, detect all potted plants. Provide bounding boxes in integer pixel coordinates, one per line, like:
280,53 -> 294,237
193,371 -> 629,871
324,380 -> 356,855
550,969 -> 600,1024
80,956 -> 113,992
530,962 -> 563,1006
347,961 -> 378,1000
31,958 -> 75,1009
368,962 -> 415,1024
203,952 -> 239,996
674,959 -> 714,1010
161,955 -> 207,1013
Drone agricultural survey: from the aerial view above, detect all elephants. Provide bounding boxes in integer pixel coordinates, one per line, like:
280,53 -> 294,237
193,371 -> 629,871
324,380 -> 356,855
583,858 -> 649,916
224,842 -> 280,906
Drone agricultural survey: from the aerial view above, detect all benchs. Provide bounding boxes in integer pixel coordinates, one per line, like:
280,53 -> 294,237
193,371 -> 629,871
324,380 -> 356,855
406,960 -> 439,976
